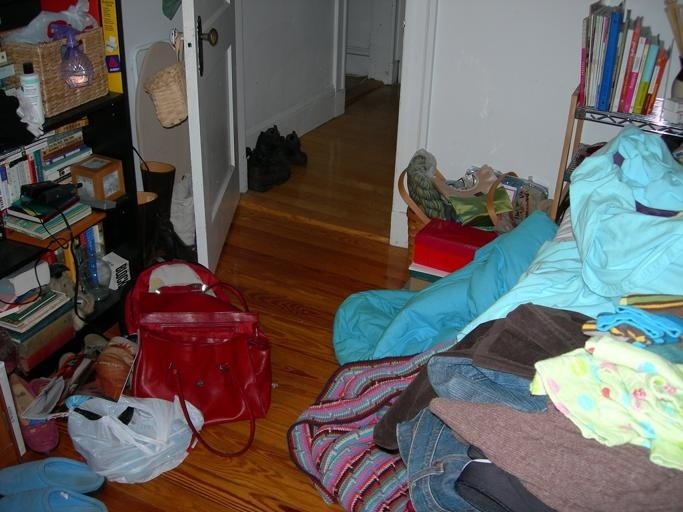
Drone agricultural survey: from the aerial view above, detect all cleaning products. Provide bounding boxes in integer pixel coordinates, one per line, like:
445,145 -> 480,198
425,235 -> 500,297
50,22 -> 93,89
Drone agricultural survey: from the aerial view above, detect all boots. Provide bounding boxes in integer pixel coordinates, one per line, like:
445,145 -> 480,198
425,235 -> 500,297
137,161 -> 195,262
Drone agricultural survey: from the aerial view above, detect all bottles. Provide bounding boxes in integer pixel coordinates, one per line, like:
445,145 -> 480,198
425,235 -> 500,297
17,62 -> 45,126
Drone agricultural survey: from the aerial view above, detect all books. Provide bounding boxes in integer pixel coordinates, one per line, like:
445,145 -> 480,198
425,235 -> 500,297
0,50 -> 94,382
18,336 -> 138,420
0,361 -> 27,456
580,0 -> 670,116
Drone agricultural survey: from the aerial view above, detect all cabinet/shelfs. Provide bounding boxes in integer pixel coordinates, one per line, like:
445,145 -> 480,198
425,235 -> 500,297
0,0 -> 144,382
551,84 -> 683,221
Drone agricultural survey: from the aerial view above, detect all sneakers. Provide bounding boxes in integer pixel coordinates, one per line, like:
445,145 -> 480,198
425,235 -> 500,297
8,373 -> 77,454
246,125 -> 306,192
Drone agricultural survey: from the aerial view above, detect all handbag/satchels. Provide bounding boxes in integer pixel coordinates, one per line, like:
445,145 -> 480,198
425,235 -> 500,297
123,259 -> 272,425
21,180 -> 83,208
143,35 -> 187,128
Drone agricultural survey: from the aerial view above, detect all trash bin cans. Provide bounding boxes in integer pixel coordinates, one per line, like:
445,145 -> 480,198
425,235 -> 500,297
169,173 -> 198,259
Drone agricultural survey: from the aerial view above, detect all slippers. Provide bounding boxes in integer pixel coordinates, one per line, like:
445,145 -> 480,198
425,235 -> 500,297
0,457 -> 106,512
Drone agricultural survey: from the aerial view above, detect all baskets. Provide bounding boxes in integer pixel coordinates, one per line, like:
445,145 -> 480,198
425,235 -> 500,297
398,167 -> 518,261
1,27 -> 109,119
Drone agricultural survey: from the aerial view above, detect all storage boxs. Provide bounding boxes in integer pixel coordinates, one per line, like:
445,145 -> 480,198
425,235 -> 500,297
70,154 -> 126,202
412,218 -> 495,273
409,263 -> 453,290
